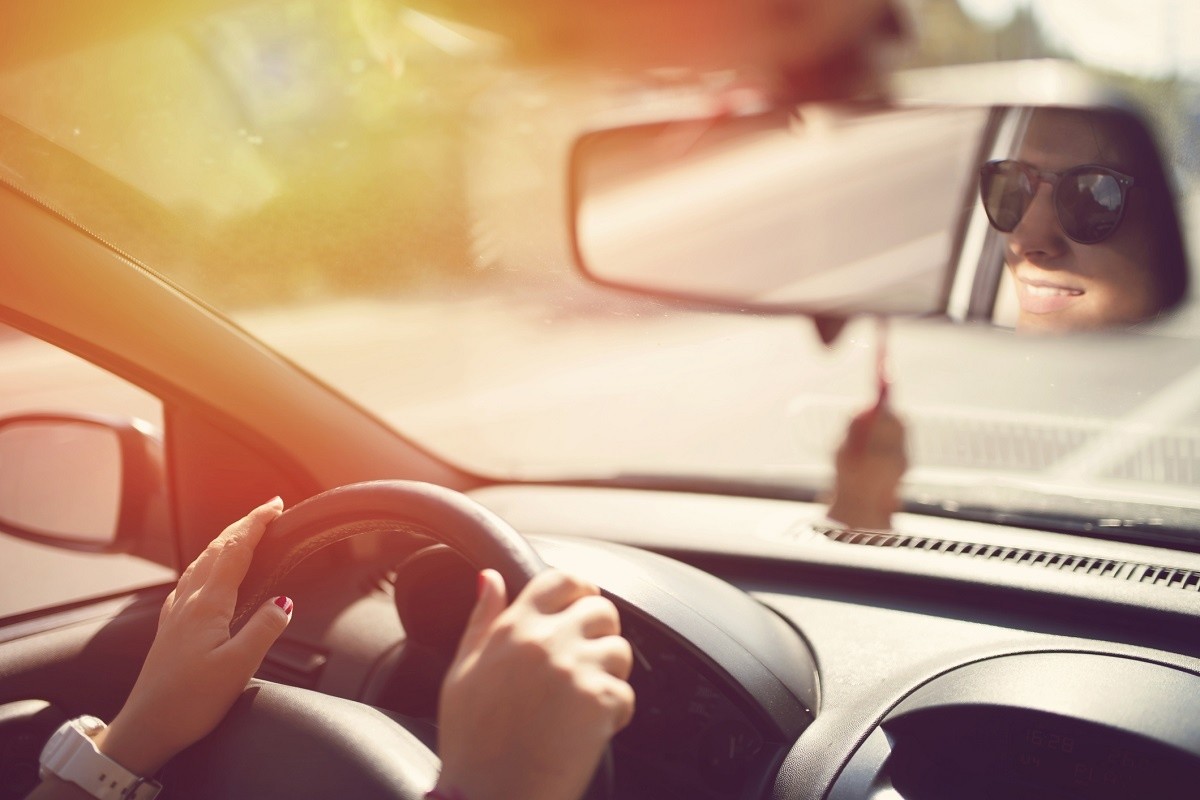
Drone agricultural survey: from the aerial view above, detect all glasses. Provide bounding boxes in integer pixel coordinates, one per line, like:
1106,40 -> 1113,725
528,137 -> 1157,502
981,156 -> 1166,245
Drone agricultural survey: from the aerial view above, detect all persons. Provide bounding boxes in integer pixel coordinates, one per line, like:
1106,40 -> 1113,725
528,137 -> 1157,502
22,494 -> 634,800
980,107 -> 1186,329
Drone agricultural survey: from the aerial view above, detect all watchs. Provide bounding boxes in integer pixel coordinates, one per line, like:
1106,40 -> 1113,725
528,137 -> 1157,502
38,714 -> 163,800
